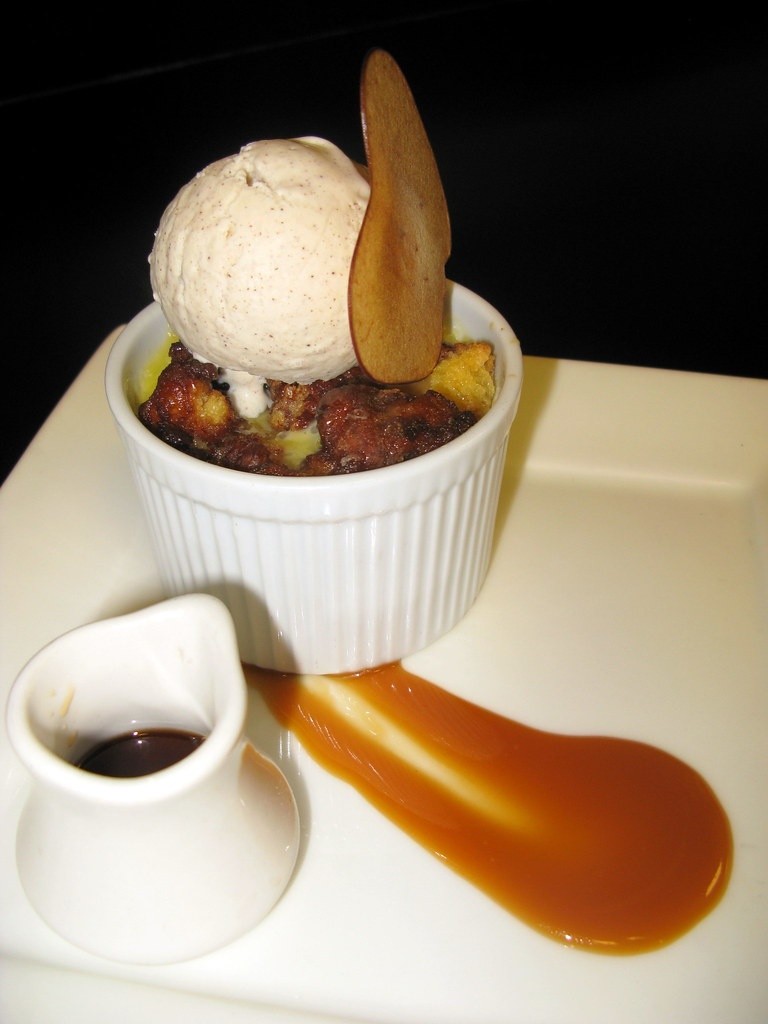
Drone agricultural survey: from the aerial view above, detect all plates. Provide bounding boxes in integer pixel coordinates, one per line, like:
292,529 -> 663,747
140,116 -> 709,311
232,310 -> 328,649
0,324 -> 768,1024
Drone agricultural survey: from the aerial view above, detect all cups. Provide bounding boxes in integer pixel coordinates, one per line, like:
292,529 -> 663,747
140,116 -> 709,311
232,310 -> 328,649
106,279 -> 525,675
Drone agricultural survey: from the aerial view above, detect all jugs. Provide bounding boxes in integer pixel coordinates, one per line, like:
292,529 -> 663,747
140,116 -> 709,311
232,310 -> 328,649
7,594 -> 300,967
